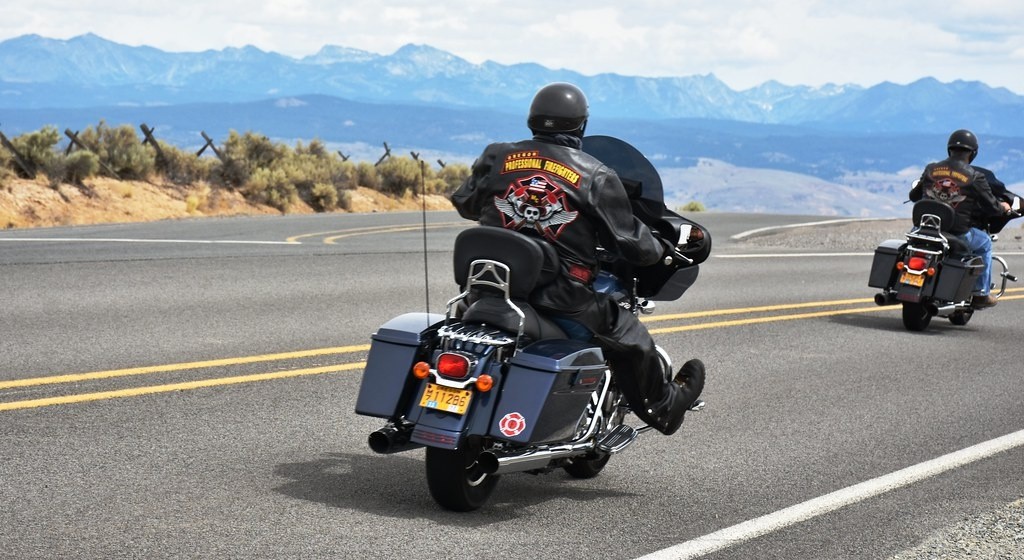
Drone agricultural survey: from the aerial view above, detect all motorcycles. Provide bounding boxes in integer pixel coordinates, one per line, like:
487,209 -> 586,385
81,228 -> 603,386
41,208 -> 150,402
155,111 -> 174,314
867,165 -> 1024,332
354,138 -> 713,515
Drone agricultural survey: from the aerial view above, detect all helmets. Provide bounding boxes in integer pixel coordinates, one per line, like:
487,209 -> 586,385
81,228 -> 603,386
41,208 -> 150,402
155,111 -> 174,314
947,129 -> 978,162
526,81 -> 589,134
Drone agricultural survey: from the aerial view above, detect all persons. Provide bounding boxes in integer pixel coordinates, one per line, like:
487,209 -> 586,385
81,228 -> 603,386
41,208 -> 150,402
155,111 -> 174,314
449,83 -> 706,438
909,130 -> 1010,308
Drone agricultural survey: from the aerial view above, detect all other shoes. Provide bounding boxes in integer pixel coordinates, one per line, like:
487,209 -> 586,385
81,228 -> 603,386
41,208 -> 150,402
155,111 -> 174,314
665,359 -> 704,436
972,295 -> 999,306
